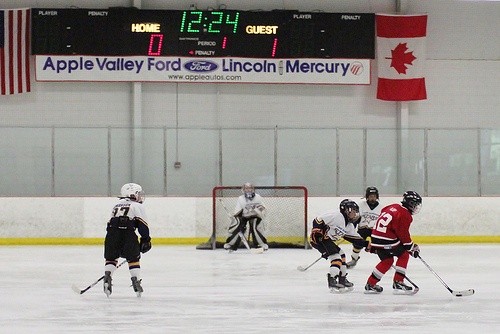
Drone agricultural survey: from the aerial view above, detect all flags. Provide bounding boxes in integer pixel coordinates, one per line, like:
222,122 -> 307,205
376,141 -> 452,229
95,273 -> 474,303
0,7 -> 30,96
375,13 -> 428,101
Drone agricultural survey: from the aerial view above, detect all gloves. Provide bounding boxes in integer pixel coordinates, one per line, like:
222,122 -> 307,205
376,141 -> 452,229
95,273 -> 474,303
311,228 -> 325,245
138,236 -> 151,254
403,241 -> 420,258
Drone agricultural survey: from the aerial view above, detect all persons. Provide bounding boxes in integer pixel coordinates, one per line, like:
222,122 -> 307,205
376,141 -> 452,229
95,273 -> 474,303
347,187 -> 384,269
309,199 -> 365,294
104,183 -> 152,297
363,191 -> 423,294
224,183 -> 268,254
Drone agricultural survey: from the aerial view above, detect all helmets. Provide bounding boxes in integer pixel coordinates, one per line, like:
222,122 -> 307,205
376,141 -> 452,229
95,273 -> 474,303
366,187 -> 379,201
243,183 -> 256,199
400,190 -> 423,214
120,182 -> 145,204
340,199 -> 360,220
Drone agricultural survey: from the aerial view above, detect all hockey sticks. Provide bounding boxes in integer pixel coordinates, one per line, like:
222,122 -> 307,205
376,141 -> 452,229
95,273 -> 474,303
71,259 -> 128,294
218,197 -> 265,254
297,239 -> 346,272
417,254 -> 475,297
391,263 -> 419,296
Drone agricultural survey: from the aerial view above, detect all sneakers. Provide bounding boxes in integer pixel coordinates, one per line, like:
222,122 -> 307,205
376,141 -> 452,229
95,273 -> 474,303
392,280 -> 413,295
338,271 -> 354,291
347,255 -> 360,268
104,271 -> 113,298
327,273 -> 348,294
130,277 -> 143,297
363,282 -> 383,294
